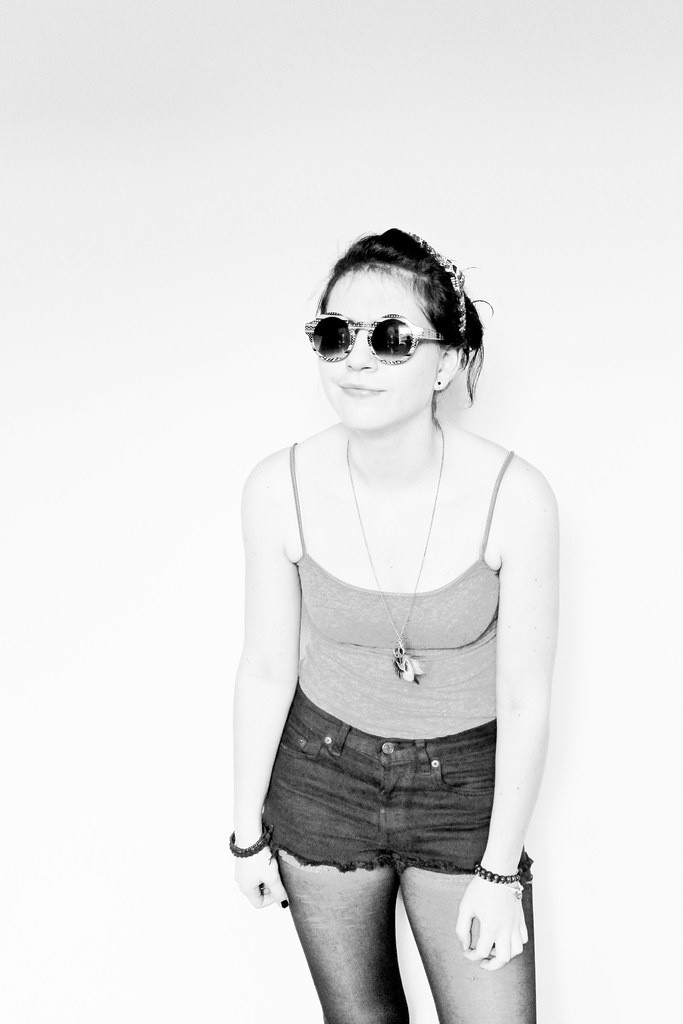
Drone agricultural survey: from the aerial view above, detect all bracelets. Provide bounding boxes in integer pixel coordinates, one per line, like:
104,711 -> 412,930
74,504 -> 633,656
229,824 -> 270,857
474,859 -> 522,884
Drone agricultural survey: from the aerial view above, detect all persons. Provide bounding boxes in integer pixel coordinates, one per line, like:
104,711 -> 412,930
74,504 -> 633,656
227,227 -> 560,1024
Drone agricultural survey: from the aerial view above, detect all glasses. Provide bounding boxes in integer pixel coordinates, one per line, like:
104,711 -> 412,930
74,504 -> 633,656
304,312 -> 444,365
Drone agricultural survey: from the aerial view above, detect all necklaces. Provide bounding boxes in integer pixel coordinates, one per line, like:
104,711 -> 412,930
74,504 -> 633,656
346,417 -> 445,685
